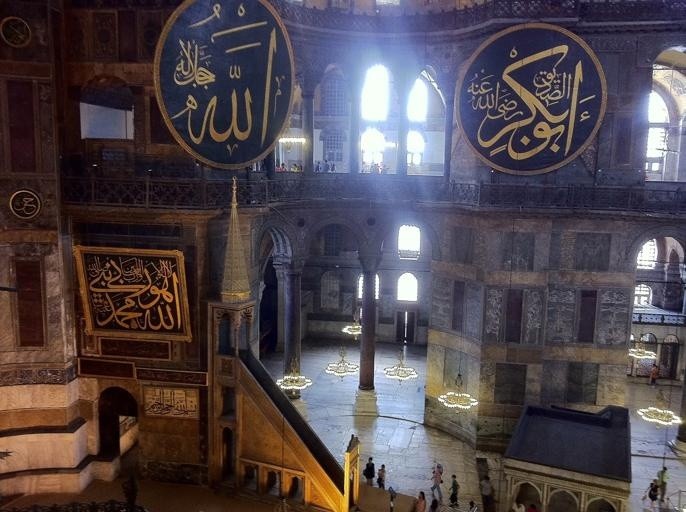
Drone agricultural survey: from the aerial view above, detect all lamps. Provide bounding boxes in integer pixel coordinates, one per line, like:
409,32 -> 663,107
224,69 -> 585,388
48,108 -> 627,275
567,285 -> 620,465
637,404 -> 684,431
624,340 -> 661,365
273,347 -> 314,402
435,250 -> 482,419
322,341 -> 360,383
339,309 -> 370,340
381,347 -> 421,388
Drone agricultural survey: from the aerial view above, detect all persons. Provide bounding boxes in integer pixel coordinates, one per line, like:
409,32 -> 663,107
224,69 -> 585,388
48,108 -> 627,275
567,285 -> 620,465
526,504 -> 539,511
414,460 -> 495,511
272,158 -> 397,174
649,363 -> 659,387
645,479 -> 660,508
510,498 -> 526,512
376,464 -> 386,489
388,486 -> 396,512
657,466 -> 671,503
364,456 -> 375,485
